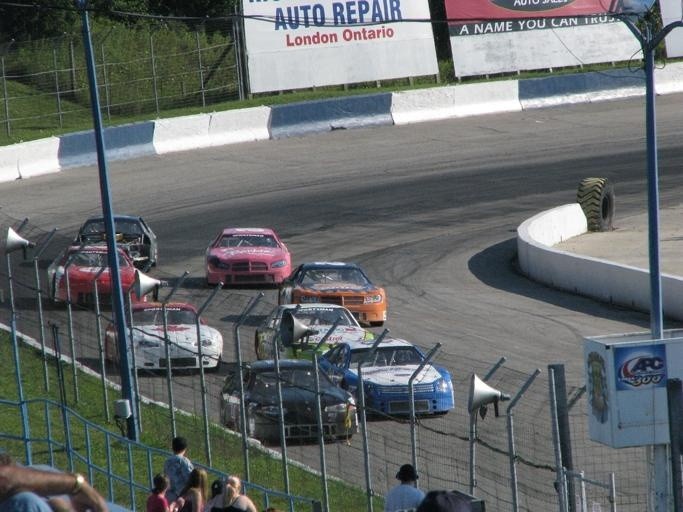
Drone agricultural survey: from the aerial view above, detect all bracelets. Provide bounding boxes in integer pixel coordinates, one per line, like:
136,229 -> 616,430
72,472 -> 84,496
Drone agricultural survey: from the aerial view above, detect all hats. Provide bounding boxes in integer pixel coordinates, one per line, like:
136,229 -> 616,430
394,462 -> 421,482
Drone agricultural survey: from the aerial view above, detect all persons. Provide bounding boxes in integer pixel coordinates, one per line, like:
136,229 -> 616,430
210,476 -> 257,511
161,436 -> 192,496
1,454 -> 106,511
180,466 -> 206,512
209,479 -> 222,500
147,474 -> 185,511
384,464 -> 423,512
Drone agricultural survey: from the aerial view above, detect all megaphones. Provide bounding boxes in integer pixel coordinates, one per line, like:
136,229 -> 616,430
134,269 -> 167,300
468,373 -> 510,413
279,311 -> 315,348
4,226 -> 35,254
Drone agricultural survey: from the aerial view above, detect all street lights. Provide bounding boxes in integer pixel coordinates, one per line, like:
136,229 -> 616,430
603,1 -> 683,512
